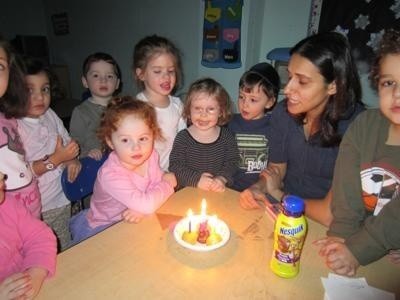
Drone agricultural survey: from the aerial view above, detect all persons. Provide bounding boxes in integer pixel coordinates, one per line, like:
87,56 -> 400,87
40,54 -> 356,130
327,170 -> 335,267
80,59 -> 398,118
239,32 -> 369,228
168,77 -> 241,191
69,34 -> 186,238
224,63 -> 281,194
1,31 -> 81,298
317,31 -> 400,277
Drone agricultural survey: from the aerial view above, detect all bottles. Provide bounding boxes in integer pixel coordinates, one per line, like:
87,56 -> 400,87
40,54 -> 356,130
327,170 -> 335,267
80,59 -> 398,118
269,195 -> 308,279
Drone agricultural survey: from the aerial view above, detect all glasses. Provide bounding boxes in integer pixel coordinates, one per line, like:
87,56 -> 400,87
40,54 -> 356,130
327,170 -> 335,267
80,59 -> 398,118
192,104 -> 218,114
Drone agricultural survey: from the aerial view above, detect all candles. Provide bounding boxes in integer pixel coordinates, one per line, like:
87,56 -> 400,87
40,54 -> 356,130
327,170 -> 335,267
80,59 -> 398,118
200,220 -> 207,232
212,226 -> 215,235
189,221 -> 191,232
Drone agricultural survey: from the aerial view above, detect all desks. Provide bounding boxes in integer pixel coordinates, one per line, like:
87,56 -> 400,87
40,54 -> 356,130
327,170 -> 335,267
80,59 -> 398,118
33,185 -> 400,300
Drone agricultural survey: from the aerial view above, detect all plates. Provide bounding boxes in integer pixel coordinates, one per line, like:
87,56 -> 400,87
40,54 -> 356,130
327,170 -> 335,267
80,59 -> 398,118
173,215 -> 231,253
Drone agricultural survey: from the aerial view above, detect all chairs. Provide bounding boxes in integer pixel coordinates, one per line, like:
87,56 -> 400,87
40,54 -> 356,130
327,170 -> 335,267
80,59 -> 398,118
267,48 -> 294,95
61,155 -> 109,217
48,65 -> 81,130
